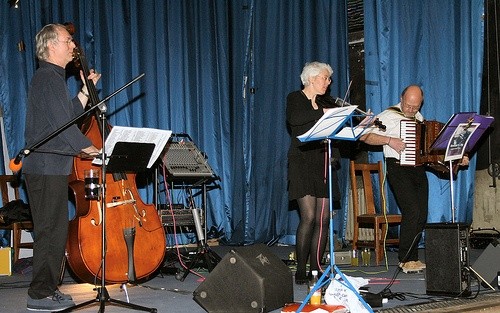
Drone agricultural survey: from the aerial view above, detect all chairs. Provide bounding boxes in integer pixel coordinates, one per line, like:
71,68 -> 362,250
349,160 -> 403,263
0,174 -> 34,264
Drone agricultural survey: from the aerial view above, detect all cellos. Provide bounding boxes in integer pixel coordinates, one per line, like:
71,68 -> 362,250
62,22 -> 166,285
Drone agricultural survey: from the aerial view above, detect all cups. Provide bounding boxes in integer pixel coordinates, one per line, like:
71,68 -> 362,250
349,250 -> 358,265
362,248 -> 371,265
84,169 -> 101,198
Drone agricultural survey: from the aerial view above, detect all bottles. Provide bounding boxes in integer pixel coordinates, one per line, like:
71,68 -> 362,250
309,270 -> 321,305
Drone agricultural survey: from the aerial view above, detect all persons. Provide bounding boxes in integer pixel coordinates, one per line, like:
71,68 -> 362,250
360,85 -> 470,275
21,23 -> 102,311
285,62 -> 377,285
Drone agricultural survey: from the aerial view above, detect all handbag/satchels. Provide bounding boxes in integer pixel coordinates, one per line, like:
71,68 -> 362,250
0,199 -> 32,225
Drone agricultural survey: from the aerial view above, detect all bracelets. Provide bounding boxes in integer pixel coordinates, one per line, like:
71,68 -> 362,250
81,89 -> 90,98
387,137 -> 391,145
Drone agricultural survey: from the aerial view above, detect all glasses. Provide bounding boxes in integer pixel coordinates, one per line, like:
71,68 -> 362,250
55,37 -> 75,47
402,97 -> 420,112
317,75 -> 332,84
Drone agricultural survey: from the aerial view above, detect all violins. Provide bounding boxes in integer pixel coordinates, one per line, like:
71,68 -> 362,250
315,94 -> 387,131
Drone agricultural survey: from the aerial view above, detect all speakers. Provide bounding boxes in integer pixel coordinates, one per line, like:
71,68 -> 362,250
474,243 -> 500,289
193,242 -> 293,313
421,226 -> 466,294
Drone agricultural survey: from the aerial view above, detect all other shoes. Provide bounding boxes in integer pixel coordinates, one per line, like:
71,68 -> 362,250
307,272 -> 330,282
295,271 -> 308,284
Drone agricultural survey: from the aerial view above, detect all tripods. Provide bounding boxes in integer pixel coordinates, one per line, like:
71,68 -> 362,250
22,72 -> 159,313
294,115 -> 374,313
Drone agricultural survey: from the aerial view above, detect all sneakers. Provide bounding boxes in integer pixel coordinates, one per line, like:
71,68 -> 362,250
54,288 -> 72,300
415,261 -> 426,269
26,293 -> 76,313
397,260 -> 423,272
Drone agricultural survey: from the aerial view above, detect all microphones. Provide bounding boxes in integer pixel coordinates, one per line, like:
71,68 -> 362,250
9,153 -> 23,172
